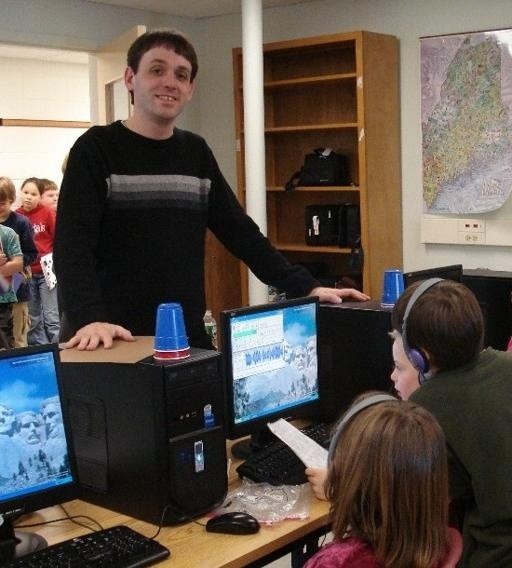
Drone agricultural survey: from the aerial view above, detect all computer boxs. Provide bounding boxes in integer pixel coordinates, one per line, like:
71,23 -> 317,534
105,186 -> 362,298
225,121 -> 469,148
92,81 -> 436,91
463,268 -> 512,352
60,346 -> 228,528
293,300 -> 402,420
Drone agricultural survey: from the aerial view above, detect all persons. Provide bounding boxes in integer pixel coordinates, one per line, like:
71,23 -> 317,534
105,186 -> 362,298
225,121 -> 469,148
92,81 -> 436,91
391,275 -> 512,567
283,334 -> 295,362
301,390 -> 453,568
387,328 -> 421,403
15,408 -> 41,447
306,333 -> 318,383
43,395 -> 63,440
15,176 -> 58,346
57,26 -> 370,354
1,174 -> 40,348
1,403 -> 16,436
1,224 -> 25,351
292,346 -> 308,375
39,179 -> 59,210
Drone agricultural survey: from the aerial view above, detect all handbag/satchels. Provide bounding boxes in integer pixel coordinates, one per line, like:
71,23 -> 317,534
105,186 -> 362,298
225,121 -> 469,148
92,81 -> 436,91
304,203 -> 359,247
300,149 -> 351,186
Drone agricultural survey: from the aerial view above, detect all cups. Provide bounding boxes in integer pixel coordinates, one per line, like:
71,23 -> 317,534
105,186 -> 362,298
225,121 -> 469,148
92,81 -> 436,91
153,302 -> 192,353
380,270 -> 407,310
153,347 -> 193,362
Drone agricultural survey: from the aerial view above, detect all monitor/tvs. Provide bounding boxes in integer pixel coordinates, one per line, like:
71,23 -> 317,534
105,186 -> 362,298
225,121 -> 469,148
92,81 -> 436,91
402,264 -> 463,290
0,342 -> 83,564
220,295 -> 323,460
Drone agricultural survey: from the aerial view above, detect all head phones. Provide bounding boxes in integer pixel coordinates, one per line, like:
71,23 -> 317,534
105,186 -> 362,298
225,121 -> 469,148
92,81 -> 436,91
401,277 -> 445,375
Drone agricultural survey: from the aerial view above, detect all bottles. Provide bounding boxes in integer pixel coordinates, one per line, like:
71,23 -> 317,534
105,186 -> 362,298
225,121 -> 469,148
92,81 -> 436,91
202,310 -> 219,351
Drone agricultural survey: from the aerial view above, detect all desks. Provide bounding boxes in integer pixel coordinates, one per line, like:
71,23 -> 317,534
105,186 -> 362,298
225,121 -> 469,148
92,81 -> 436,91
11,415 -> 348,568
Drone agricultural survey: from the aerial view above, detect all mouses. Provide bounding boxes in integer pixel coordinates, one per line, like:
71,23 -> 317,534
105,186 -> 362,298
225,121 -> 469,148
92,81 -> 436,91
206,512 -> 260,535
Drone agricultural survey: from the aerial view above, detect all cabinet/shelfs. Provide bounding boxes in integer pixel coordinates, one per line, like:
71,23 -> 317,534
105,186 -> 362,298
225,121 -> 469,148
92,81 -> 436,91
231,31 -> 403,308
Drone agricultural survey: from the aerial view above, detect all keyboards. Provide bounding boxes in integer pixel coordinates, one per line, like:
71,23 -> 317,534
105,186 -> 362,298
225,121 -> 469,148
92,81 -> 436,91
236,419 -> 332,486
0,525 -> 171,568
